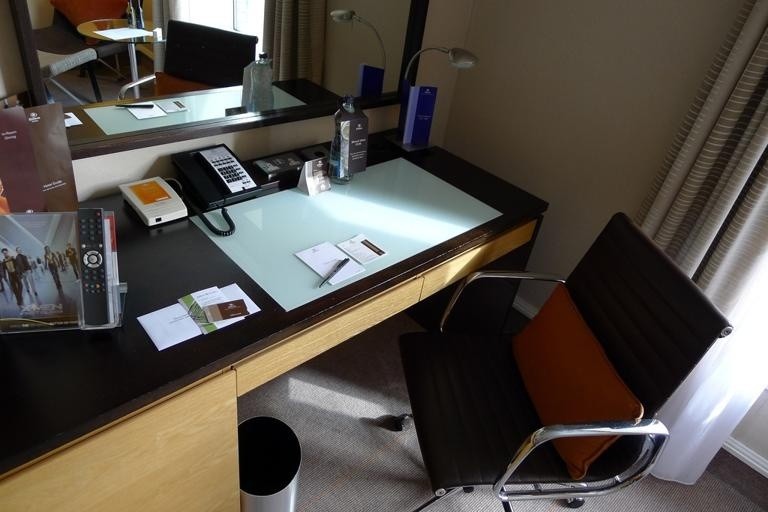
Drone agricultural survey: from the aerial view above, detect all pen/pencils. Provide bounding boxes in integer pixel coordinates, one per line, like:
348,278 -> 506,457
116,104 -> 153,108
320,258 -> 349,288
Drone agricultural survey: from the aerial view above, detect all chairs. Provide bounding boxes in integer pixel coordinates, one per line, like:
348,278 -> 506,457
394,213 -> 734,511
117,20 -> 258,102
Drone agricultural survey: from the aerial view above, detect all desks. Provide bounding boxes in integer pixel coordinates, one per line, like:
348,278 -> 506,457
0,129 -> 549,510
64,78 -> 343,144
78,18 -> 169,97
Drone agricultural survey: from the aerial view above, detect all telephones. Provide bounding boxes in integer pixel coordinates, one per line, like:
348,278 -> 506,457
171,143 -> 262,210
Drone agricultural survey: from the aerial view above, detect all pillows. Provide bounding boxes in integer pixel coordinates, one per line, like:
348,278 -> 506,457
510,283 -> 647,482
50,0 -> 129,46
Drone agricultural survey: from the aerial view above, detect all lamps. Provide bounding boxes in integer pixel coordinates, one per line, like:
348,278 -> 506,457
330,10 -> 386,96
386,45 -> 480,152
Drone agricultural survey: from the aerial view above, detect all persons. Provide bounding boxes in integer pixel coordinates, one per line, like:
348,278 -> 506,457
0,243 -> 80,305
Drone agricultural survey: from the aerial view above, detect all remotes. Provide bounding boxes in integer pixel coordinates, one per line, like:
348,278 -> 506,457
75,205 -> 113,329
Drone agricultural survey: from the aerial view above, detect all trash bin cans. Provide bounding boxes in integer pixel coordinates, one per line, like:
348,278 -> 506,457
238,415 -> 303,512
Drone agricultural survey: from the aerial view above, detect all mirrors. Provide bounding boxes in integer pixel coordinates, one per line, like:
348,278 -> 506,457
7,0 -> 431,162
